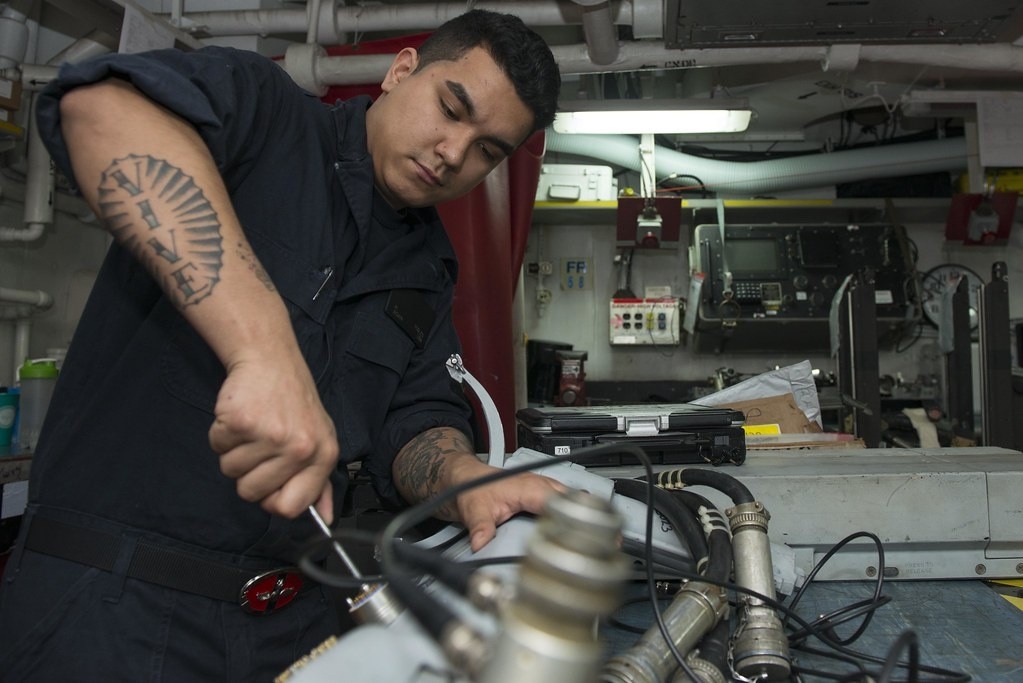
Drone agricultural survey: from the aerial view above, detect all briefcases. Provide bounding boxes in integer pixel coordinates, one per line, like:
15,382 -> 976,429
516,399 -> 746,467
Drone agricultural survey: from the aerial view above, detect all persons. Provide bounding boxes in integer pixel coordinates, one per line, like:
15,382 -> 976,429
0,8 -> 609,683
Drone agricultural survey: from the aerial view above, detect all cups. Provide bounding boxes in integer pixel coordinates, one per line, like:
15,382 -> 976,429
0,393 -> 18,445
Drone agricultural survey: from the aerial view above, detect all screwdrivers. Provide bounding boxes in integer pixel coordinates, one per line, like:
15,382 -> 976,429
306,503 -> 370,594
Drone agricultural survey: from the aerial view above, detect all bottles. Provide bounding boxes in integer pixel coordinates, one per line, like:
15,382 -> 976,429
16,359 -> 58,448
